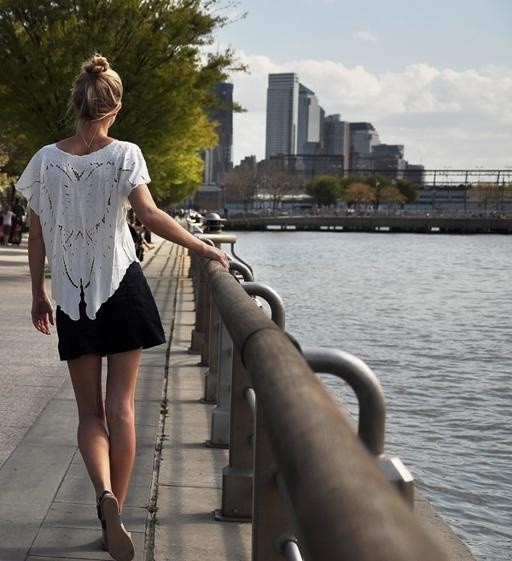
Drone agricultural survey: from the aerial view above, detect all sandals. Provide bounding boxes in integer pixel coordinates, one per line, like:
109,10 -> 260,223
97,491 -> 137,560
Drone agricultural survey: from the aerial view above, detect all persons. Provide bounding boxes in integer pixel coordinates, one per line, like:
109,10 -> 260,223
14,54 -> 229,561
0,198 -> 188,250
